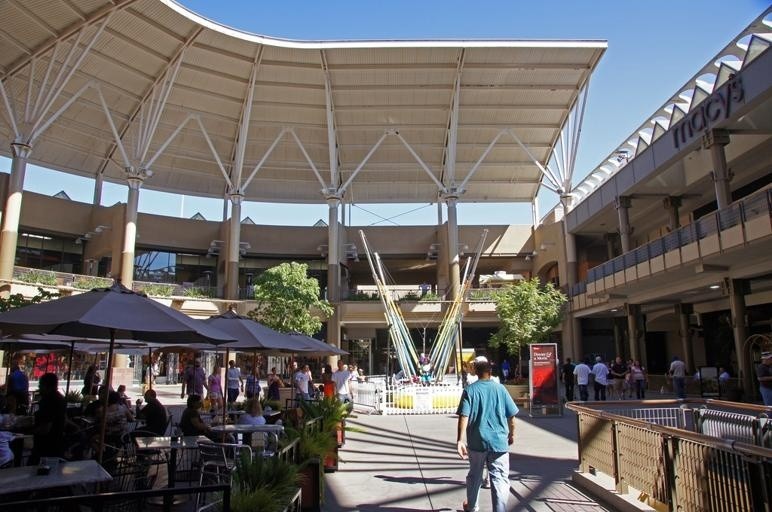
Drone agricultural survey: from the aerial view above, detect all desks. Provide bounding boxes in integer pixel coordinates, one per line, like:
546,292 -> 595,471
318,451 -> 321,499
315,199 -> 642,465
0,400 -> 283,512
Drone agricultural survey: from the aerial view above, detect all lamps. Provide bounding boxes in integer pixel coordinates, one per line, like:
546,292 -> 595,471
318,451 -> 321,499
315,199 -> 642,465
205,238 -> 226,260
317,244 -> 327,263
74,225 -> 111,246
457,243 -> 469,257
342,243 -> 360,264
524,241 -> 555,263
425,243 -> 442,262
239,241 -> 251,262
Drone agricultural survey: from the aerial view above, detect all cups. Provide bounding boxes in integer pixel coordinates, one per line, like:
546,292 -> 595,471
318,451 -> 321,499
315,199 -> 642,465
37,467 -> 50,475
171,436 -> 178,441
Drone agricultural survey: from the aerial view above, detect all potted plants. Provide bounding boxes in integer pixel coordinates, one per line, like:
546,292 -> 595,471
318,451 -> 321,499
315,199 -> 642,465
204,395 -> 367,512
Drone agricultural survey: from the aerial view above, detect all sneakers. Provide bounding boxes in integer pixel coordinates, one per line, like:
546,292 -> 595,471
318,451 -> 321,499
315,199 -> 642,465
462,500 -> 477,512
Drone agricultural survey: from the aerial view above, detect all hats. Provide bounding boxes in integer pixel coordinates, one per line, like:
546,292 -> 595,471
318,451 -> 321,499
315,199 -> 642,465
595,356 -> 601,361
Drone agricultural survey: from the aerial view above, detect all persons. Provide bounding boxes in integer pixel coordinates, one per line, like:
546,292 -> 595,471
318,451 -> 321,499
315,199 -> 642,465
757,351 -> 772,420
461,361 -> 468,387
348,364 -> 354,371
0,346 -> 352,468
352,363 -> 359,377
558,352 -> 733,402
464,356 -> 502,489
418,281 -> 431,297
394,369 -> 405,384
453,355 -> 523,511
490,358 -> 498,376
420,357 -> 432,386
502,358 -> 512,383
